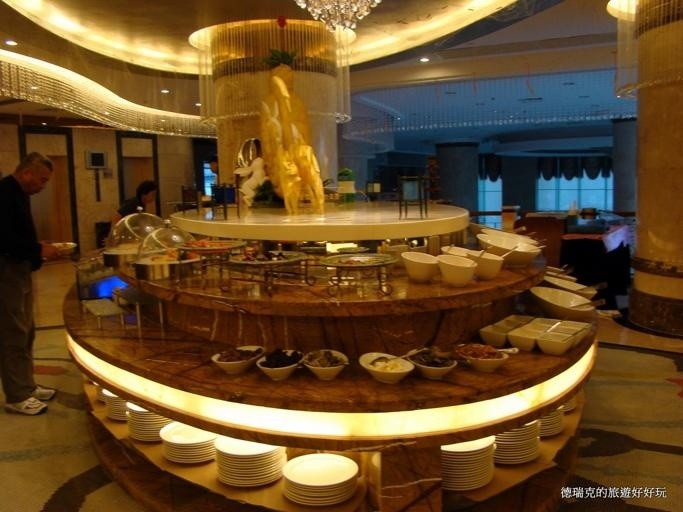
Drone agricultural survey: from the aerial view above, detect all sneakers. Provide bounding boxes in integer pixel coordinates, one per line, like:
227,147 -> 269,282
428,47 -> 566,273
4,397 -> 49,415
29,385 -> 56,400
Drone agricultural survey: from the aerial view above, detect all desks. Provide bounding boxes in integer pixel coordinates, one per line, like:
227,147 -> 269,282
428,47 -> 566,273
561,222 -> 630,312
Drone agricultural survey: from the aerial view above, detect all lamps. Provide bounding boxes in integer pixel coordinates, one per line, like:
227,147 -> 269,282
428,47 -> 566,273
292,0 -> 383,33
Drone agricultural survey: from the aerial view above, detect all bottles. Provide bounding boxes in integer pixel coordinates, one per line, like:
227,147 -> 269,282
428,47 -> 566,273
337,160 -> 356,203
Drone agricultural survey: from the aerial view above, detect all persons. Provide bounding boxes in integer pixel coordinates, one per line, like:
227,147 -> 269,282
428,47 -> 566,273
208,155 -> 219,185
0,150 -> 63,414
113,182 -> 158,225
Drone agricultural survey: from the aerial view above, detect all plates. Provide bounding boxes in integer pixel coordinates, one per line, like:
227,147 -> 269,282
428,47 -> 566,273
441,435 -> 496,491
215,434 -> 288,487
540,405 -> 564,436
159,421 -> 217,463
102,388 -> 129,421
93,381 -> 105,402
558,395 -> 576,411
127,401 -> 172,441
494,420 -> 540,464
281,454 -> 359,507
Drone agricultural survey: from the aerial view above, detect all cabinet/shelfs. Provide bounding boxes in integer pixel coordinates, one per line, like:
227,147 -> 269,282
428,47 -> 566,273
63,200 -> 596,512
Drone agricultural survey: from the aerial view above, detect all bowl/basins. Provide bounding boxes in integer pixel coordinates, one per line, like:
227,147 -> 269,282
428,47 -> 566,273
478,314 -> 592,356
50,242 -> 77,254
400,245 -> 504,288
211,345 -> 351,383
358,344 -> 510,386
469,220 -> 541,269
531,264 -> 599,318
